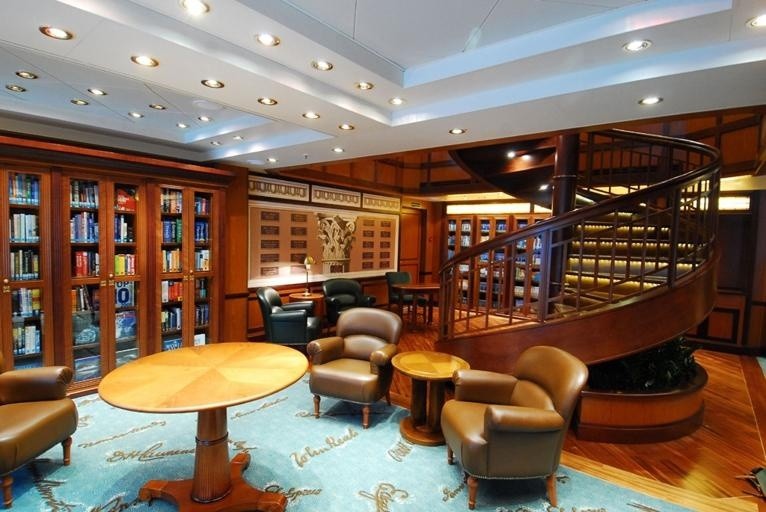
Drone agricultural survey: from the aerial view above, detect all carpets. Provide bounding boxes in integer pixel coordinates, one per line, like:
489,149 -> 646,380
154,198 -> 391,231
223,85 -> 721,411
0,372 -> 757,512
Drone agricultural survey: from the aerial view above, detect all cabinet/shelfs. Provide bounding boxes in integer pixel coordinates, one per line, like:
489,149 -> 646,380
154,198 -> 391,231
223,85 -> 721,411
0,160 -> 237,395
445,213 -> 550,313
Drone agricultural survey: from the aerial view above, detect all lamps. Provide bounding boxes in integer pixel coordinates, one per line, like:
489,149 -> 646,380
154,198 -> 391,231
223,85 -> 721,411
302,255 -> 314,296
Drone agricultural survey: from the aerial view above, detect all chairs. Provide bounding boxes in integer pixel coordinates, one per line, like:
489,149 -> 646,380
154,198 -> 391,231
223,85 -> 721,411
308,308 -> 403,428
253,270 -> 426,351
1,366 -> 76,508
441,346 -> 590,511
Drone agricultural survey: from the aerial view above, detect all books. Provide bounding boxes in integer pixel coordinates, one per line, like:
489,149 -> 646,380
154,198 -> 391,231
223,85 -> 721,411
72,280 -> 140,381
11,287 -> 43,369
161,218 -> 182,242
8,214 -> 40,243
161,248 -> 183,273
113,187 -> 137,242
7,174 -> 39,205
194,278 -> 209,346
161,190 -> 183,212
70,180 -> 99,244
9,250 -> 39,281
75,251 -> 100,277
114,254 -> 135,276
162,280 -> 184,302
194,196 -> 209,243
160,308 -> 182,350
446,218 -> 546,311
194,247 -> 210,272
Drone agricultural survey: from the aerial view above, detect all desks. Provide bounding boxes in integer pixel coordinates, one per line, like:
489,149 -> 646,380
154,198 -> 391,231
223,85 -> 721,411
96,340 -> 309,510
391,351 -> 470,447
